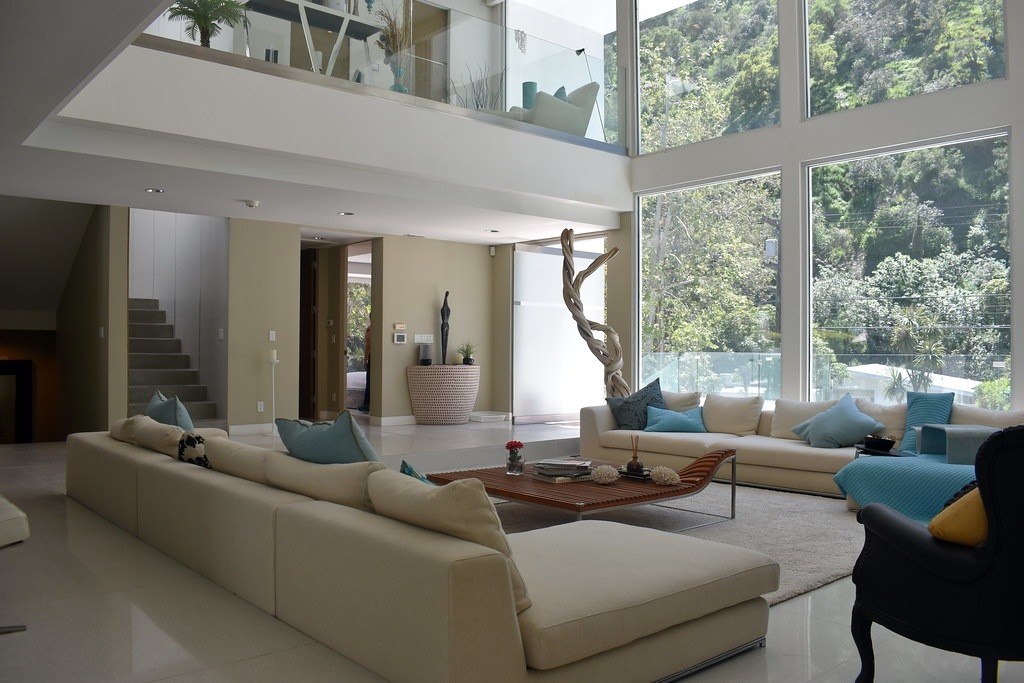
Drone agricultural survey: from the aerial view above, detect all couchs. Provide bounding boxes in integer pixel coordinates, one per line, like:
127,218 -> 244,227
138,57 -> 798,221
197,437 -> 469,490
578,405 -> 1024,511
509,82 -> 600,138
66,432 -> 780,683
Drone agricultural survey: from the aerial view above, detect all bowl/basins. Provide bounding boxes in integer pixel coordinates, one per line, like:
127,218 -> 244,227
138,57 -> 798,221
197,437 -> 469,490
865,437 -> 895,452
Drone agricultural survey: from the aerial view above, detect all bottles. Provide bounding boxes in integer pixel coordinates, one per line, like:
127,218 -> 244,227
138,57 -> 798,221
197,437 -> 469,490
627,457 -> 643,473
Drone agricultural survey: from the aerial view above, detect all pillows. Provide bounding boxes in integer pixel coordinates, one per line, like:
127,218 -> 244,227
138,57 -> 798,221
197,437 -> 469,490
110,377 -> 1024,615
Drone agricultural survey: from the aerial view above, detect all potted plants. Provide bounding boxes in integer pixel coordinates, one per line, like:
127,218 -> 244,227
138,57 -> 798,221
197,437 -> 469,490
864,433 -> 897,451
457,339 -> 482,364
373,0 -> 410,94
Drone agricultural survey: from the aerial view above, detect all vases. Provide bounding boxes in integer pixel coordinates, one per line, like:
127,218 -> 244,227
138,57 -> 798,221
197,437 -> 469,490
505,453 -> 525,475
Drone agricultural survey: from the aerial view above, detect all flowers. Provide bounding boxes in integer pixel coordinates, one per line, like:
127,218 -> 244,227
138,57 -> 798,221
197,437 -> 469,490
504,440 -> 524,452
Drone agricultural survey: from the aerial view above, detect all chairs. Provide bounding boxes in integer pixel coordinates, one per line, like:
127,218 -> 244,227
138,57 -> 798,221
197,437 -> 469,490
850,425 -> 1024,683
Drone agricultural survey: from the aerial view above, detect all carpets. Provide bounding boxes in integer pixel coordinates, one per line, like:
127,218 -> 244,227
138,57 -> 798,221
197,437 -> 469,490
423,456 -> 867,608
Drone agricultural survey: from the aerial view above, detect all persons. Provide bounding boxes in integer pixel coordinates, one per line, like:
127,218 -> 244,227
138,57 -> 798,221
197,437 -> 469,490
358,313 -> 371,414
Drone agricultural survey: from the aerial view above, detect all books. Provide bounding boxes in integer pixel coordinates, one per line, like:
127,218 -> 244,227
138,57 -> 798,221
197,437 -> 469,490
532,457 -> 595,483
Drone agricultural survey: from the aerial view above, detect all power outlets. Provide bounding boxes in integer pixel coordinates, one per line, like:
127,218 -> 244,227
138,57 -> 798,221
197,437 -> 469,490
269,330 -> 276,341
257,402 -> 264,412
331,334 -> 335,343
219,329 -> 224,340
414,334 -> 433,344
97,327 -> 103,339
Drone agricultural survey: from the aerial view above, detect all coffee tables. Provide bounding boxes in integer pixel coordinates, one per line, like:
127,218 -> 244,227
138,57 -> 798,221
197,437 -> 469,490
425,449 -> 736,533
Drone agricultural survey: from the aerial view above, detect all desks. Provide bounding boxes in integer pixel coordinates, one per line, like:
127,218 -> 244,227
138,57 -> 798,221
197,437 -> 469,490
239,0 -> 381,76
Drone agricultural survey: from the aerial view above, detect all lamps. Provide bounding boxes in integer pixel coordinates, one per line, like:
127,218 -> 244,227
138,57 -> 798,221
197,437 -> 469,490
575,48 -> 608,143
